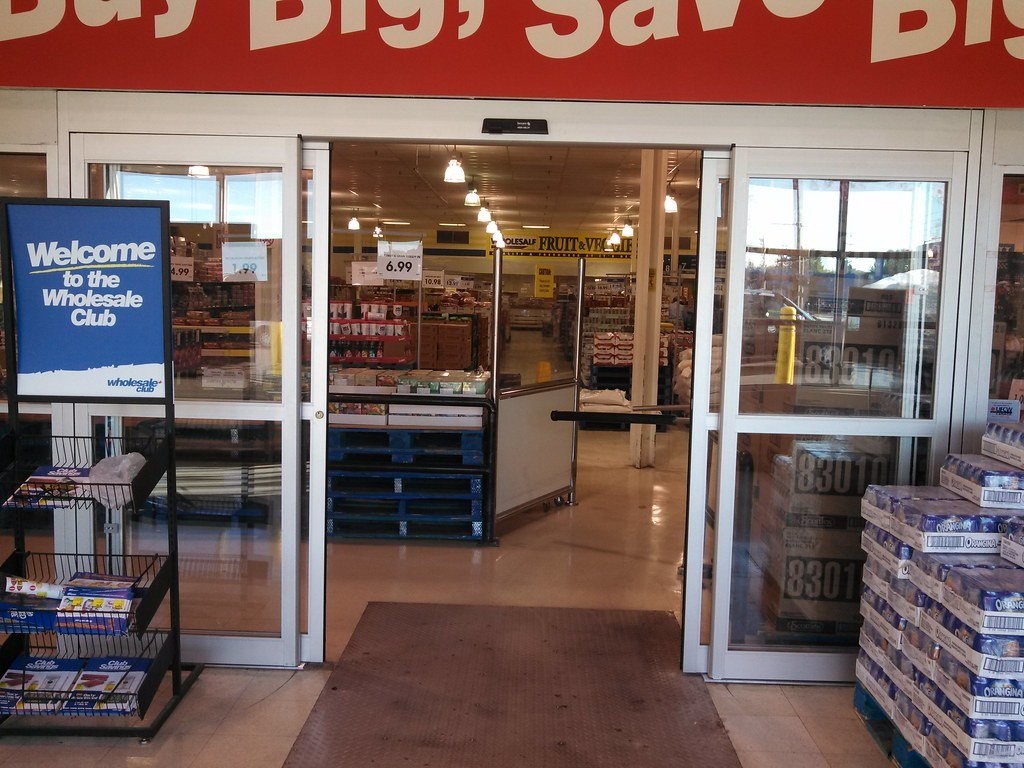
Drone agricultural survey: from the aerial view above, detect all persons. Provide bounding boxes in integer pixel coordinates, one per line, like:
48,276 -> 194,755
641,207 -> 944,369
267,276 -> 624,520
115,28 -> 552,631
993,286 -> 1017,332
669,296 -> 688,320
559,294 -> 578,339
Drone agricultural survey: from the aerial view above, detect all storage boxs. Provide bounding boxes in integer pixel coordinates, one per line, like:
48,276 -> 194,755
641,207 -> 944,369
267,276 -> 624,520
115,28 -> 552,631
301,323 -> 491,427
749,286 -> 1024,644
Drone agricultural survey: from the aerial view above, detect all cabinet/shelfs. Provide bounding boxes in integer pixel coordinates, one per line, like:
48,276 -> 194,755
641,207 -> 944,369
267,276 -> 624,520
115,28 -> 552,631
170,236 -> 255,377
551,281 -> 675,433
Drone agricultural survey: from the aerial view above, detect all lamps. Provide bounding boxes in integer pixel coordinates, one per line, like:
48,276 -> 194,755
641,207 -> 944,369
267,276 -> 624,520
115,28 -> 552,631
493,227 -> 502,242
348,212 -> 360,231
372,226 -> 383,238
486,212 -> 497,233
622,216 -> 633,238
606,233 -> 612,245
477,197 -> 491,222
464,176 -> 481,206
664,187 -> 678,214
496,235 -> 505,248
611,227 -> 620,244
444,145 -> 466,183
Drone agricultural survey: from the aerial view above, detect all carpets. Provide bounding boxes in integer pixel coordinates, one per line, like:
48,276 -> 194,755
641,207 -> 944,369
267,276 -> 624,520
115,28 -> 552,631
282,601 -> 743,768
